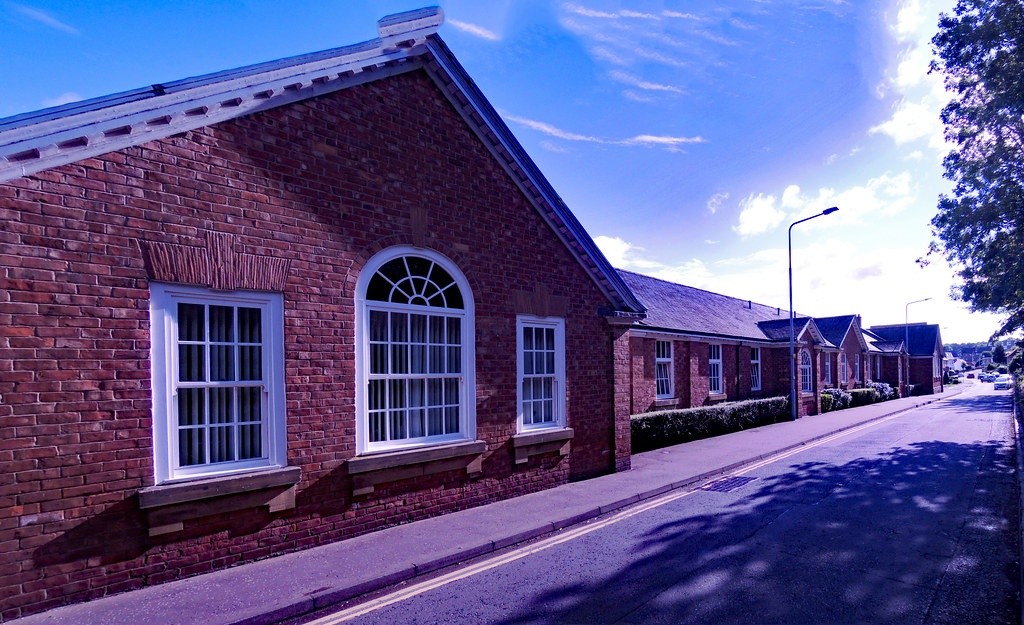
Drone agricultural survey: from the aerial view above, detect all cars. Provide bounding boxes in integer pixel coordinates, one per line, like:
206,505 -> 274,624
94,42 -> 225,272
978,373 -> 1013,388
967,373 -> 975,379
958,372 -> 964,377
993,377 -> 1010,390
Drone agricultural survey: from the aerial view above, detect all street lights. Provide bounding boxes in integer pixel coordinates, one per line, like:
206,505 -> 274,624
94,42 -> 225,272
905,297 -> 935,397
971,350 -> 978,362
788,206 -> 840,421
